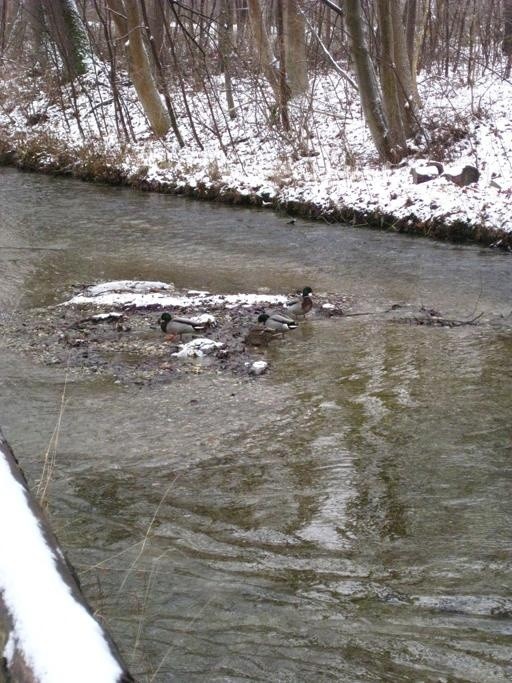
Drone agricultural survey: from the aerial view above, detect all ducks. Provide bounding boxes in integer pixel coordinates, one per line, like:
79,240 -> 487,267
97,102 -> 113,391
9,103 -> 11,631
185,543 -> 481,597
159,313 -> 204,340
256,314 -> 298,336
286,286 -> 313,320
